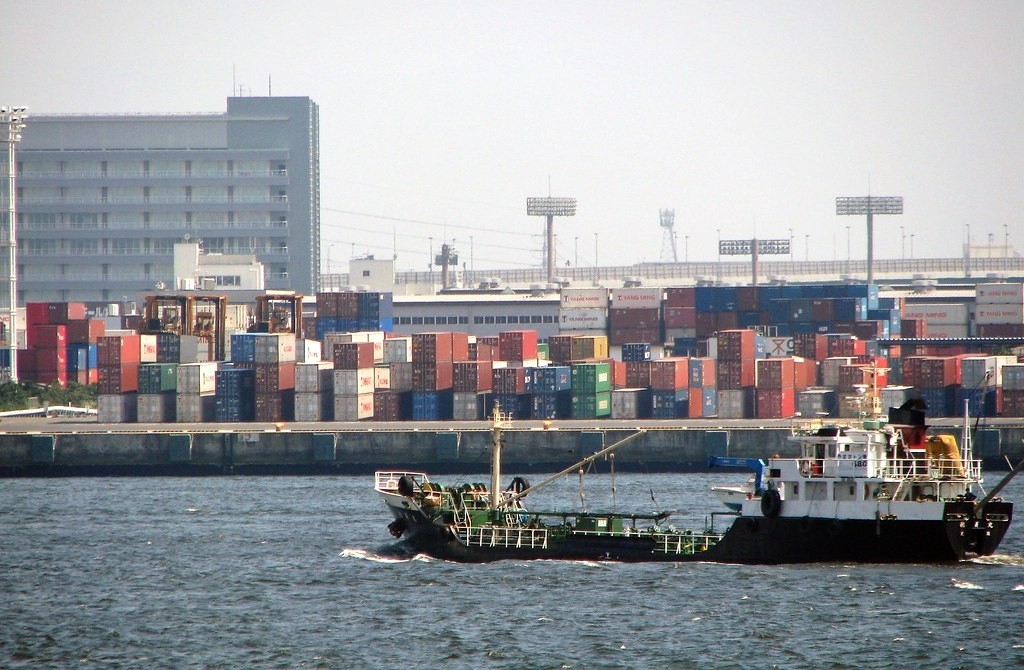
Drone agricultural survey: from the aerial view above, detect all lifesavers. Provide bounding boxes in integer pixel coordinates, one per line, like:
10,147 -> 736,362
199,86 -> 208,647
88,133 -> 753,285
511,477 -> 530,498
397,475 -> 414,496
760,490 -> 781,518
800,460 -> 813,474
420,482 -> 488,497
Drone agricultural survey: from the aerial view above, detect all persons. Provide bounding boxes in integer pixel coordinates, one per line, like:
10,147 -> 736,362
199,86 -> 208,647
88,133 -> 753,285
964,488 -> 977,501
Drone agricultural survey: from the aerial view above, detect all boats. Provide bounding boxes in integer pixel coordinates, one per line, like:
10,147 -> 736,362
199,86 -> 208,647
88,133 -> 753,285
375,353 -> 1024,568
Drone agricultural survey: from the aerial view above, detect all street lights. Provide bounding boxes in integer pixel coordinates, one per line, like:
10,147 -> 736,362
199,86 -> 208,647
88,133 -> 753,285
966,224 -> 970,259
988,233 -> 992,258
574,237 -> 578,267
716,229 -> 720,262
351,243 -> 356,259
910,234 -> 915,258
327,244 -> 334,274
788,228 -> 794,262
594,232 -> 598,266
685,235 -> 688,262
847,226 -> 849,259
805,235 -> 810,261
428,237 -> 433,272
1004,224 -> 1007,256
0,105 -> 30,387
470,236 -> 473,270
